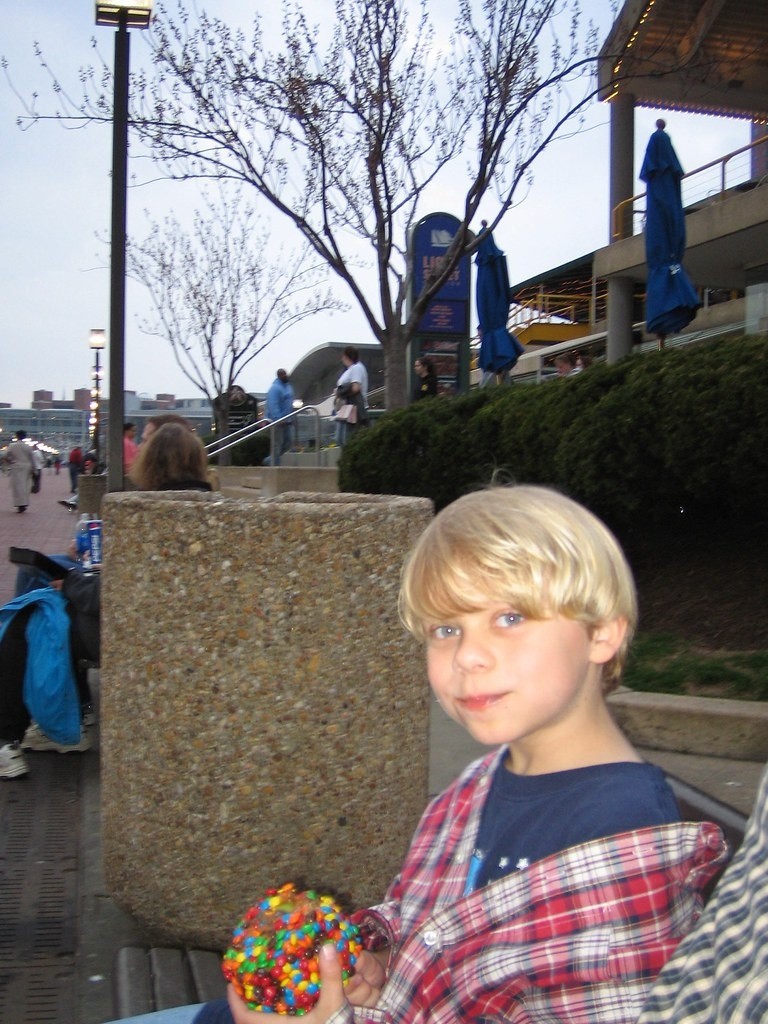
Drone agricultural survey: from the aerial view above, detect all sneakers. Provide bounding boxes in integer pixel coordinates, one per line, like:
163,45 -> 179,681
21,723 -> 92,752
0,745 -> 30,778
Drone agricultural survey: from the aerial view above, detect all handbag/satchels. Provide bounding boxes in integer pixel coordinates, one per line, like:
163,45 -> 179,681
334,402 -> 357,425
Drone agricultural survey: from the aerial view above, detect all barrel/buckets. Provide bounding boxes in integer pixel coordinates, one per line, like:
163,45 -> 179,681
307,440 -> 315,447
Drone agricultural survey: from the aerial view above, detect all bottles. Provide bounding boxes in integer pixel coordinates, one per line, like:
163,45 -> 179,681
76,513 -> 95,576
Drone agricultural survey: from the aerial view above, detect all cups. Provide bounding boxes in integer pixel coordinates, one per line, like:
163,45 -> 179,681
86,520 -> 102,564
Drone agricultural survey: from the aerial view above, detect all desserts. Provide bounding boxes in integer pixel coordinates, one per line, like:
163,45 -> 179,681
220,884 -> 364,1015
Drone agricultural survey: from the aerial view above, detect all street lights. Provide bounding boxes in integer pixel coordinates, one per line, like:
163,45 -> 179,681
89,328 -> 106,460
90,0 -> 162,489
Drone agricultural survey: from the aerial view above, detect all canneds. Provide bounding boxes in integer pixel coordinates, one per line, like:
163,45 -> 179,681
86,519 -> 102,564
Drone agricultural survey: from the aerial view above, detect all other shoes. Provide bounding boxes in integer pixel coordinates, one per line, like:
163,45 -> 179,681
18,505 -> 26,513
59,499 -> 79,511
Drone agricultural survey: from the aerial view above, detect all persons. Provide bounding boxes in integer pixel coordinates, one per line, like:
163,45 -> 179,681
331,345 -> 372,449
553,349 -> 580,380
265,369 -> 296,466
641,762 -> 768,1022
476,326 -> 513,386
16,415 -> 198,725
0,429 -> 105,513
122,423 -> 143,472
413,358 -> 437,400
0,413 -> 213,778
108,486 -> 730,1022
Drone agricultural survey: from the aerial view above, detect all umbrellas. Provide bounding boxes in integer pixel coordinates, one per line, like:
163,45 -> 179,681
477,220 -> 525,385
640,118 -> 704,349
